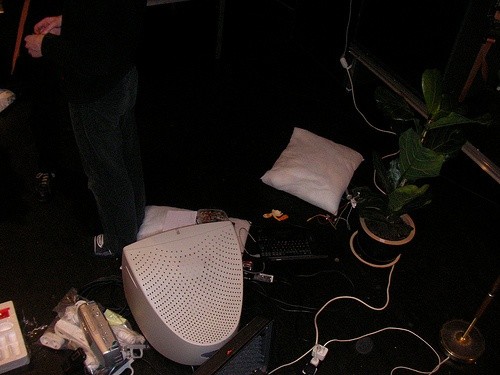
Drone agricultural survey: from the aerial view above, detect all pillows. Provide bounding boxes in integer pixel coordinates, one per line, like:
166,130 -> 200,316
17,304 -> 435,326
136,205 -> 252,253
261,127 -> 364,215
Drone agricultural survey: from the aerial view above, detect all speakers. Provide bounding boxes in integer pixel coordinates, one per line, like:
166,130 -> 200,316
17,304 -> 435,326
121,221 -> 243,365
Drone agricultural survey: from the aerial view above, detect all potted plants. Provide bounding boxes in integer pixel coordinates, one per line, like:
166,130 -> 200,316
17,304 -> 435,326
350,69 -> 491,267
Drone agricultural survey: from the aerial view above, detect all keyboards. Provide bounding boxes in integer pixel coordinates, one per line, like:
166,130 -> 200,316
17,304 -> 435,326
258,240 -> 329,261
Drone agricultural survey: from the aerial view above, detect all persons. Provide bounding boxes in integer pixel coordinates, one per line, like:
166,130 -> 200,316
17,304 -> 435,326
0,0 -> 62,203
23,0 -> 146,254
